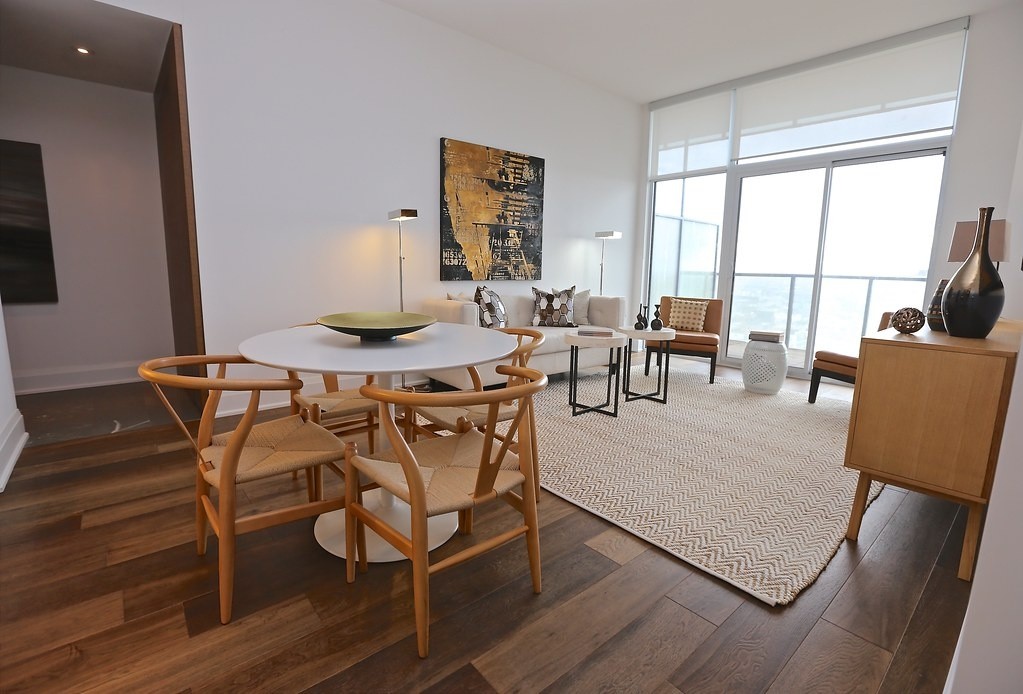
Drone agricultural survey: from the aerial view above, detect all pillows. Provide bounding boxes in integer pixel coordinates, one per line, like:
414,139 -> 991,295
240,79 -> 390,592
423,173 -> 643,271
667,298 -> 710,332
551,287 -> 591,328
529,285 -> 577,326
474,286 -> 508,331
447,290 -> 482,326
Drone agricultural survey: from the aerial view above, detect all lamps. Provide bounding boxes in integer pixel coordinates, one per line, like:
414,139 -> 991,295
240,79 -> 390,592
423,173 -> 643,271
388,209 -> 423,388
595,230 -> 622,295
950,219 -> 1011,272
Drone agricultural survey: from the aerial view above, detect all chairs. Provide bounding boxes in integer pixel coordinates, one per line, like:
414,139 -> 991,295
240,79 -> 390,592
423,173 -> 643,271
808,312 -> 896,404
413,329 -> 543,534
355,366 -> 550,657
644,296 -> 723,384
287,320 -> 380,503
137,354 -> 368,626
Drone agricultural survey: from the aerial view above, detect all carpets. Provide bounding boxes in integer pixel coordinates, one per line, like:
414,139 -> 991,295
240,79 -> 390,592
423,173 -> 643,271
395,360 -> 887,607
15,381 -> 201,448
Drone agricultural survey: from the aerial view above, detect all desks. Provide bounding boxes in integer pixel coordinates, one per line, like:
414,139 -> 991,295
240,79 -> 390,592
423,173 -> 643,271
564,330 -> 627,417
618,327 -> 675,404
844,315 -> 1023,582
238,325 -> 518,564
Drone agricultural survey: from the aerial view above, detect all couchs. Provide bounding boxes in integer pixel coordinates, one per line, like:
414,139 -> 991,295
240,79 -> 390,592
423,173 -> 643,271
422,296 -> 626,392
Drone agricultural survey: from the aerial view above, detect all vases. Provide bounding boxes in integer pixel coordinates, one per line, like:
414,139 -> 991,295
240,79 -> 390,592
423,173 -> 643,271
941,206 -> 1005,340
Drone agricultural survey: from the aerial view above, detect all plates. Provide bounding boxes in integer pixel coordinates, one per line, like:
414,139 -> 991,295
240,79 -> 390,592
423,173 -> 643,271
316,311 -> 438,341
